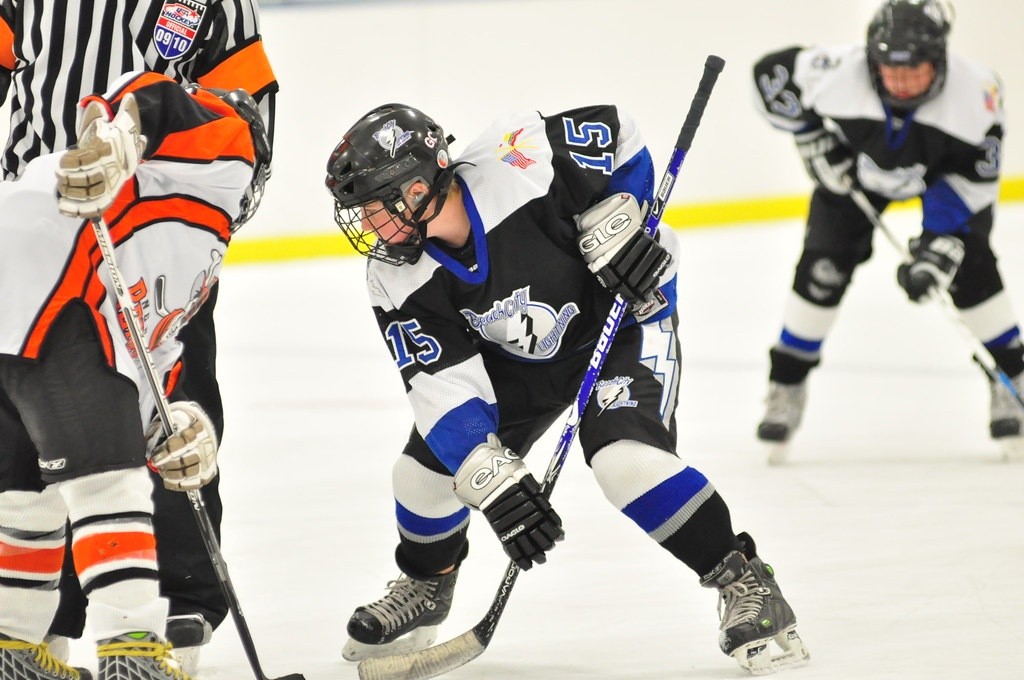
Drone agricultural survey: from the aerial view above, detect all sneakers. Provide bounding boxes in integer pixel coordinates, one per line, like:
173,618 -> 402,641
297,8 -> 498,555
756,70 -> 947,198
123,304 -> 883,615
759,377 -> 813,461
92,635 -> 197,680
162,596 -> 216,676
985,349 -> 1024,451
0,630 -> 93,680
698,532 -> 810,674
343,537 -> 469,662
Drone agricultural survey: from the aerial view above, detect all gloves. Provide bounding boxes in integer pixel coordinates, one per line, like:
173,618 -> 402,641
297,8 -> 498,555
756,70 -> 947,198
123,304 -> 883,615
897,235 -> 968,307
572,192 -> 676,302
792,123 -> 857,196
144,401 -> 219,491
53,91 -> 148,220
452,433 -> 565,571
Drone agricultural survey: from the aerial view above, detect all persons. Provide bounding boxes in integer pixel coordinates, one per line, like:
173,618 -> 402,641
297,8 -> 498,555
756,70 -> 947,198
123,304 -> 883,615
753,0 -> 1024,442
0,0 -> 278,654
325,103 -> 810,676
0,70 -> 272,680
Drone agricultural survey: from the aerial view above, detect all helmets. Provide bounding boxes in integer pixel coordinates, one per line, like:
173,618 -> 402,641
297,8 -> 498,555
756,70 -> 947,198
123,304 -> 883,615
325,103 -> 460,266
865,0 -> 951,108
183,82 -> 271,235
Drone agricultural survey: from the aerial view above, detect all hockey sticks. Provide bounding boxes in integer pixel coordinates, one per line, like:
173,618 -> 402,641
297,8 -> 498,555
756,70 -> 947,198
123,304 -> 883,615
356,55 -> 729,679
849,177 -> 1024,408
89,210 -> 307,680
